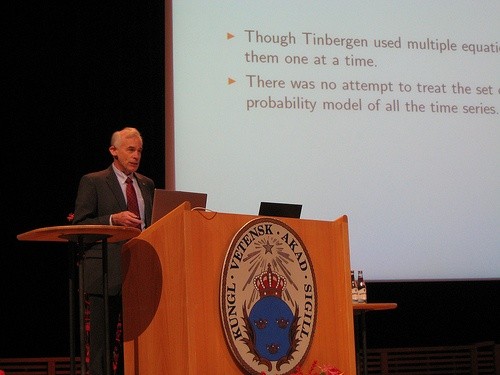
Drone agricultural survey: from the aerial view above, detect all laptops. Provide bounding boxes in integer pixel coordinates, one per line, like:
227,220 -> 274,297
259,202 -> 302,218
151,189 -> 207,227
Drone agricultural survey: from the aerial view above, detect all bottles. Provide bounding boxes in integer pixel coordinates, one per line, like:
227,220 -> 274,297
351,270 -> 358,302
357,271 -> 366,303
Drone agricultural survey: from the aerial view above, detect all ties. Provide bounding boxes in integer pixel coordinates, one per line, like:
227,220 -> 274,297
125,177 -> 141,232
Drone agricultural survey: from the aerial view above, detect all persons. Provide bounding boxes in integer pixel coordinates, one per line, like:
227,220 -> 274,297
72,126 -> 156,375
